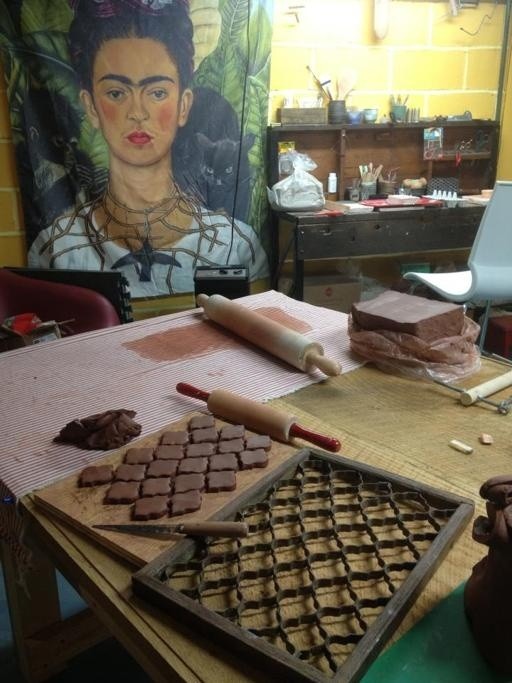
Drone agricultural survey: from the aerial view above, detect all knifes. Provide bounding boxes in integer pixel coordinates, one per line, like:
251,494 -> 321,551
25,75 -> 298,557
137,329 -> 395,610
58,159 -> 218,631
91,520 -> 251,539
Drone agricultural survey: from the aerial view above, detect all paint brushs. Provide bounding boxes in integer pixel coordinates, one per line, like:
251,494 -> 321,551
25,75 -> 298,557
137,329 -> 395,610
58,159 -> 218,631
306,65 -> 356,100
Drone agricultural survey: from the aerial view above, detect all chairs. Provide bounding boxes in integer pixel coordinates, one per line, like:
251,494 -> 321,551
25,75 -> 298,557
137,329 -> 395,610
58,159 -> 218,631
404,181 -> 511,354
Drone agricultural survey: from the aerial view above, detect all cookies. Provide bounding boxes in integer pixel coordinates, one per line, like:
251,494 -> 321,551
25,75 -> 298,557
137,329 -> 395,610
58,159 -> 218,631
77,414 -> 272,520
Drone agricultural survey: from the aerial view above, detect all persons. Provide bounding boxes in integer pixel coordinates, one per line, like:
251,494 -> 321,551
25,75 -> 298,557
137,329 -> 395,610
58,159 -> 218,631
24,4 -> 275,303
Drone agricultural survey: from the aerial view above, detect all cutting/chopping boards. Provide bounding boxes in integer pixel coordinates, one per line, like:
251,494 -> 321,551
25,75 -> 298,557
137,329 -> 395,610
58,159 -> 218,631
31,409 -> 302,569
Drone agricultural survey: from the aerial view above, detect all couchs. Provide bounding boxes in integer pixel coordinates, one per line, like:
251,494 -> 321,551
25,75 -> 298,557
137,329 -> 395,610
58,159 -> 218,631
2,270 -> 119,337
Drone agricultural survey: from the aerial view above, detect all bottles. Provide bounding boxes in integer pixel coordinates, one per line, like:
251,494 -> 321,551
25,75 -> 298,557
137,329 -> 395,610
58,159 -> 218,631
327,172 -> 337,192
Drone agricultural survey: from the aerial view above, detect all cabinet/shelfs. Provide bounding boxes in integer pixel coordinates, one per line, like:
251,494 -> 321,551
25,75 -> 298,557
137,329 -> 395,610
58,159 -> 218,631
267,121 -> 499,192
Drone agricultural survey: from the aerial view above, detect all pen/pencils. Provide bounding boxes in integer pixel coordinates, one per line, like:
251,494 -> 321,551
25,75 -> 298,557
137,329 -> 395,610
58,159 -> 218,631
391,93 -> 409,105
358,161 -> 401,182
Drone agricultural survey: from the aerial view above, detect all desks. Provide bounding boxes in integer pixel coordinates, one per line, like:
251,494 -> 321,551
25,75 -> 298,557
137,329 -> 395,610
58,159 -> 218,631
279,193 -> 485,302
0,292 -> 512,682
